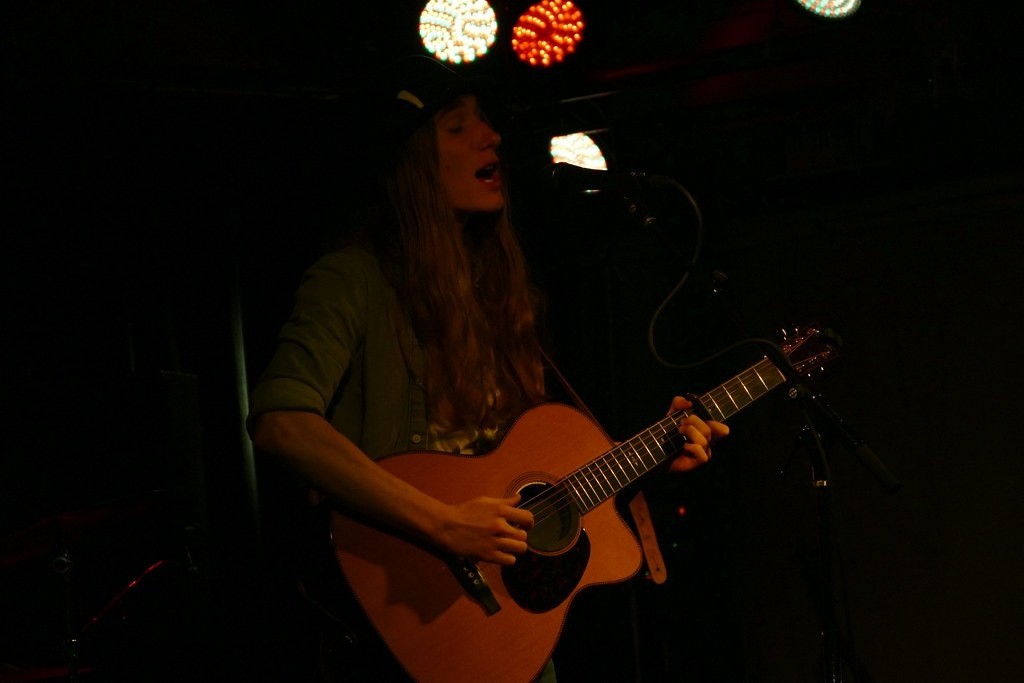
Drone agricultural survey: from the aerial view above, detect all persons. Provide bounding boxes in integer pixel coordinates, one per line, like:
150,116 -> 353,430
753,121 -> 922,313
250,55 -> 731,682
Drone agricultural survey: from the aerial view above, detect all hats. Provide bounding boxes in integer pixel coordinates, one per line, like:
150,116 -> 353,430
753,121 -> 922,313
365,52 -> 470,161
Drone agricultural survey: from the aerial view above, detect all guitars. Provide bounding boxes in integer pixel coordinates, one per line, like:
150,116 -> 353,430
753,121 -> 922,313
329,323 -> 833,683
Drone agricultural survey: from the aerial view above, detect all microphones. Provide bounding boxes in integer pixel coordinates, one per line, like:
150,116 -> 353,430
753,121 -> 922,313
553,161 -> 677,199
